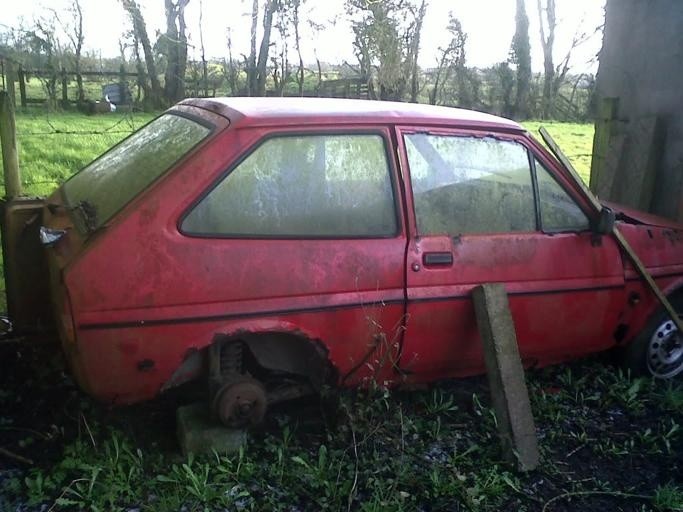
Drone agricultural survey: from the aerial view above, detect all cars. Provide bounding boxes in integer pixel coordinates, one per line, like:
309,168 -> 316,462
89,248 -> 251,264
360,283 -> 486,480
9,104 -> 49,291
1,96 -> 682,430
